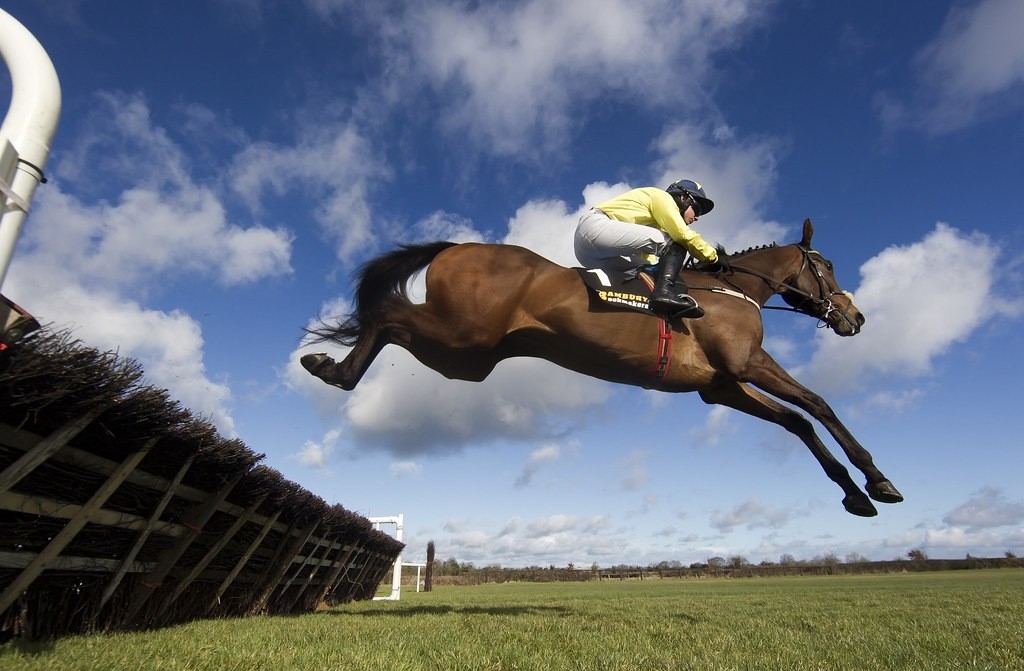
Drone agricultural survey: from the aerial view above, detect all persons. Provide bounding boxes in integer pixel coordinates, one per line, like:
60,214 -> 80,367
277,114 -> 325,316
573,180 -> 729,318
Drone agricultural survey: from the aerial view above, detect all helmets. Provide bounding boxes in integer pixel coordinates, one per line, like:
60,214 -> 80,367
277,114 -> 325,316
666,180 -> 714,216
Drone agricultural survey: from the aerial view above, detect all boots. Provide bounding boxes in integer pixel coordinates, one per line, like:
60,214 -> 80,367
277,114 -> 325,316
651,241 -> 705,319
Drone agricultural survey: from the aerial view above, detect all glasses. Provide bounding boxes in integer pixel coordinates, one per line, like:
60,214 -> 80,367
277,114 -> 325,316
677,185 -> 702,217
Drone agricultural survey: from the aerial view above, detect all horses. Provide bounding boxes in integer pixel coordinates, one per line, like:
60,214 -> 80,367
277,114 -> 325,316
297,215 -> 904,519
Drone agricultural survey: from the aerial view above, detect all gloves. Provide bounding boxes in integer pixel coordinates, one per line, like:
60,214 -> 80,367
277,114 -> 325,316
713,254 -> 734,274
